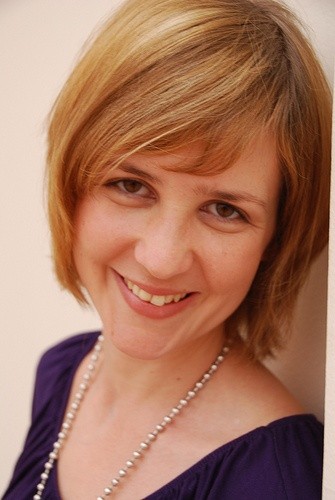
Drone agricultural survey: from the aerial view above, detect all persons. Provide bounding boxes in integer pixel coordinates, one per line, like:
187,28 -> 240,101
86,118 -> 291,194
0,0 -> 334,499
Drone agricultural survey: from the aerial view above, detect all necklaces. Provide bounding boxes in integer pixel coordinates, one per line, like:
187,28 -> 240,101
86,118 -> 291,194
33,328 -> 238,499
